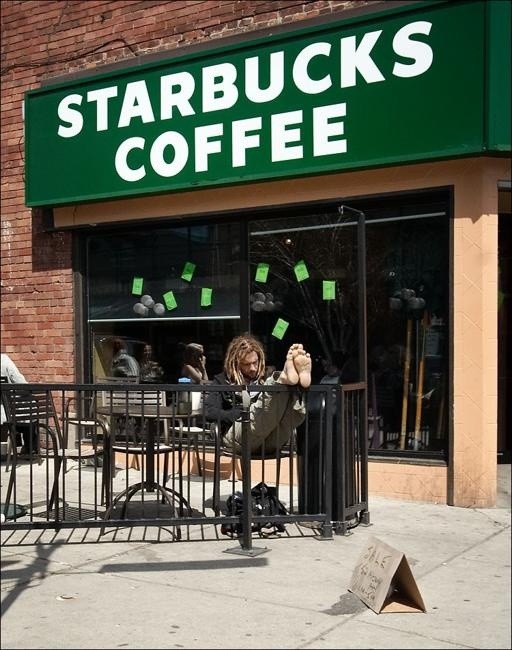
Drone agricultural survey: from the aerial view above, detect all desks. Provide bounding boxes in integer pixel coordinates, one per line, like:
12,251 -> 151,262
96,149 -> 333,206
96,403 -> 204,539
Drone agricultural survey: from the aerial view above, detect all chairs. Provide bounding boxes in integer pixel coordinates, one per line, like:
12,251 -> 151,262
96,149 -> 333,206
93,390 -> 184,514
211,423 -> 301,518
0,384 -> 104,533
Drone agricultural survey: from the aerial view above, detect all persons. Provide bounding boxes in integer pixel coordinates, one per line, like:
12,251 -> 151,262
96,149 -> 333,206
174,343 -> 207,412
0,351 -> 41,459
202,336 -> 313,456
109,337 -> 167,385
311,349 -> 346,417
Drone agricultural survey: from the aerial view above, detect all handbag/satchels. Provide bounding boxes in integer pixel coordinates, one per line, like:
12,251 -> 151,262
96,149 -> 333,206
219,483 -> 287,534
